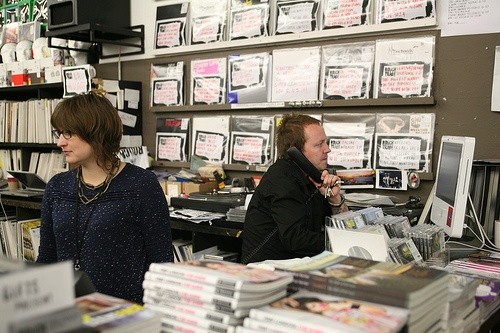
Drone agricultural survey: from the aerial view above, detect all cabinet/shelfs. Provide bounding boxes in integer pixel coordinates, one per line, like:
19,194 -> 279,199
0,22 -> 441,251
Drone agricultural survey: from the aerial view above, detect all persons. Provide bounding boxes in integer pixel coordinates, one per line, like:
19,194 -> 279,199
35,91 -> 174,305
199,260 -> 278,282
242,112 -> 349,264
285,297 -> 397,333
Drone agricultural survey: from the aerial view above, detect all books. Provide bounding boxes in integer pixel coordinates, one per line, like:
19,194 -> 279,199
0,260 -> 160,333
0,210 -> 41,264
172,238 -> 192,263
0,149 -> 69,184
142,251 -> 500,333
325,206 -> 448,268
204,251 -> 239,263
2,21 -> 41,43
0,96 -> 67,144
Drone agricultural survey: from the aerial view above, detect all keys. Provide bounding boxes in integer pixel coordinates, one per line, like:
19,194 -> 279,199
324,181 -> 345,199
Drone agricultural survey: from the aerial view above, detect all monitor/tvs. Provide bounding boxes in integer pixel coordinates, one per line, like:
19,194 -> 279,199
429,134 -> 476,243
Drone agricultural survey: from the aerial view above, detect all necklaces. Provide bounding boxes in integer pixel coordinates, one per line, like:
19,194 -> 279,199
74,160 -> 115,271
75,157 -> 120,205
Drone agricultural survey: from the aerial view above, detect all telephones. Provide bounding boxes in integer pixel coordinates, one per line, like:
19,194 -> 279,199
286,146 -> 323,183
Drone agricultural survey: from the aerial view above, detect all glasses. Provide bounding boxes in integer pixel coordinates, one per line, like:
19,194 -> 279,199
51,128 -> 75,139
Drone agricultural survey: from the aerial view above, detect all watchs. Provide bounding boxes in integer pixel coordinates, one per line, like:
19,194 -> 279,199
328,194 -> 348,207
